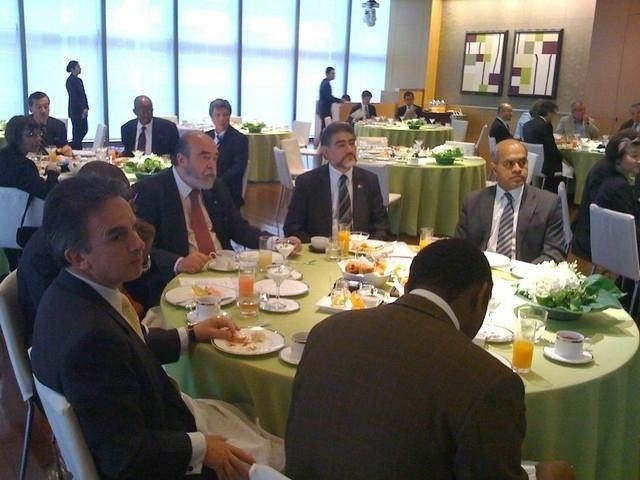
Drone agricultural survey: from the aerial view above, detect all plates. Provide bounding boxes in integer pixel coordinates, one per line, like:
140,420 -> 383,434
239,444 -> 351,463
265,270 -> 303,281
278,346 -> 300,365
436,157 -> 455,165
475,324 -> 515,344
308,245 -> 325,253
186,310 -> 230,322
210,325 -> 286,357
165,285 -> 236,308
543,345 -> 592,365
314,289 -> 387,312
135,171 -> 159,178
235,250 -> 283,271
259,298 -> 300,313
254,278 -> 310,297
483,251 -> 510,267
210,262 -> 240,272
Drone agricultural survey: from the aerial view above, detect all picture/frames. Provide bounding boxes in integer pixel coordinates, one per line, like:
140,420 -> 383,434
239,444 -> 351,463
508,28 -> 564,98
460,30 -> 509,97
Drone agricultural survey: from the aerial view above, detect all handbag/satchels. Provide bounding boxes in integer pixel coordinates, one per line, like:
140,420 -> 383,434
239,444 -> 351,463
16,193 -> 40,248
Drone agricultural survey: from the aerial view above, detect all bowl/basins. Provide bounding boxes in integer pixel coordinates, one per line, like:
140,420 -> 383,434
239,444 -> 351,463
336,259 -> 391,288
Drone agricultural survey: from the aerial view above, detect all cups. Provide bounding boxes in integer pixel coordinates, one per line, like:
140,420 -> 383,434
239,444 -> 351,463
235,295 -> 259,322
327,239 -> 342,262
555,331 -> 591,358
337,224 -> 350,258
511,320 -> 537,373
349,232 -> 369,261
259,236 -> 273,268
275,240 -> 297,265
518,306 -> 548,343
290,331 -> 309,359
331,289 -> 347,308
196,296 -> 221,321
310,237 -> 328,248
419,227 -> 434,249
267,264 -> 295,308
238,264 -> 255,300
209,250 -> 236,267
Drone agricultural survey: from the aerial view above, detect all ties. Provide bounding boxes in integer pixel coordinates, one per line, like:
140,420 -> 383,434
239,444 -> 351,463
337,174 -> 352,233
121,294 -> 180,392
495,193 -> 514,258
365,106 -> 370,119
137,127 -> 145,154
216,135 -> 222,150
189,190 -> 216,259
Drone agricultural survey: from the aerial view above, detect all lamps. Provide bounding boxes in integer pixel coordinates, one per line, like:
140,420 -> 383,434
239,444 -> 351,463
364,0 -> 380,27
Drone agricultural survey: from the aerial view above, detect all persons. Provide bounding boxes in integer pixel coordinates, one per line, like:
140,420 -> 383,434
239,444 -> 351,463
204,98 -> 249,196
555,100 -> 601,141
453,138 -> 567,266
283,122 -> 389,242
514,101 -> 543,139
489,103 -> 514,144
347,90 -> 377,126
28,91 -> 73,156
17,160 -> 159,321
28,174 -> 256,480
66,61 -> 89,143
132,131 -> 302,314
619,98 -> 640,131
282,237 -> 576,480
524,100 -> 576,196
121,96 -> 179,156
0,116 -> 61,201
394,92 -> 423,122
317,66 -> 342,130
596,133 -> 640,252
568,127 -> 637,264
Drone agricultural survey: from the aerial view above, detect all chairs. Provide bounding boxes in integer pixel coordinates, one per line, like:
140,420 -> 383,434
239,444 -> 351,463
485,151 -> 539,189
584,203 -> 640,315
355,162 -> 401,242
272,146 -> 298,218
553,181 -> 599,280
89,124 -> 109,153
288,121 -> 312,169
32,361 -> 114,480
1,187 -> 43,267
280,137 -> 313,181
445,124 -> 488,156
488,135 -> 497,165
521,143 -> 568,198
1,268 -> 57,480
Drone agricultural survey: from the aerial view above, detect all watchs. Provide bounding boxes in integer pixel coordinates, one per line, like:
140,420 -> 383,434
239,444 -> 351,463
186,321 -> 197,341
